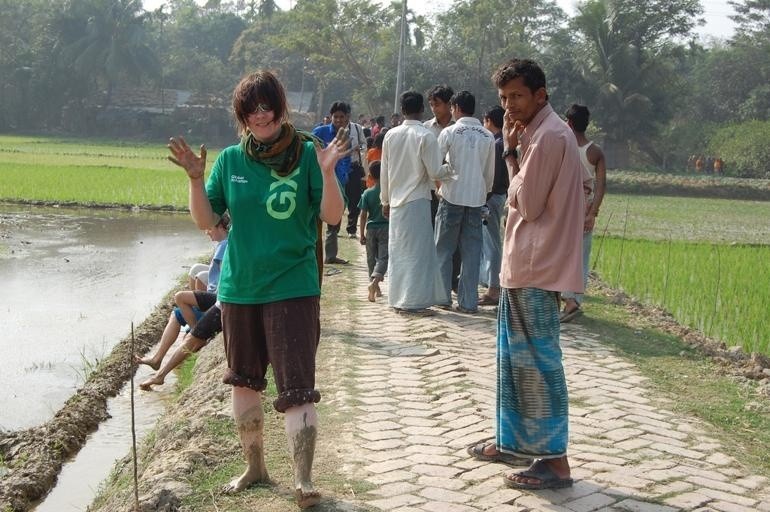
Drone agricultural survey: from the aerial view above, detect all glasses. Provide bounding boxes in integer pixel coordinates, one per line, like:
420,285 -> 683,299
250,102 -> 272,113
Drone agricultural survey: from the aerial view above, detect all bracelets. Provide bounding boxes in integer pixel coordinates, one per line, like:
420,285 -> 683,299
188,172 -> 205,179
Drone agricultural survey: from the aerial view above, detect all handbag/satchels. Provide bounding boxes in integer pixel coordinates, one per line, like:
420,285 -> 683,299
350,160 -> 364,181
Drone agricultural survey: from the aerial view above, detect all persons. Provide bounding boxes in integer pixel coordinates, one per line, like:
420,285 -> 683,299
133,216 -> 232,391
313,83 -> 606,323
466,57 -> 587,490
165,68 -> 360,510
685,153 -> 722,177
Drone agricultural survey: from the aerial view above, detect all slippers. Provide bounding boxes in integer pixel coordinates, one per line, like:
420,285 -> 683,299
504,460 -> 574,490
468,442 -> 530,466
324,268 -> 342,276
393,295 -> 499,316
560,304 -> 582,323
